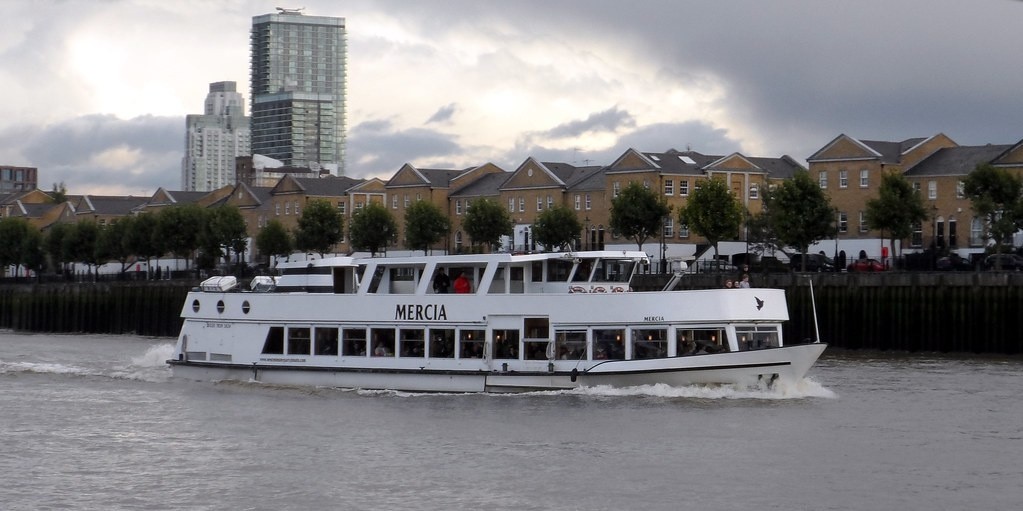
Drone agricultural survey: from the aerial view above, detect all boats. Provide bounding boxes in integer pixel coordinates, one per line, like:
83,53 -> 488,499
164,226 -> 830,395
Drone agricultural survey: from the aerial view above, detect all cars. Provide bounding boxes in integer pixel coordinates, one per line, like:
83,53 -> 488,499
936,256 -> 975,272
848,258 -> 890,273
983,253 -> 1023,273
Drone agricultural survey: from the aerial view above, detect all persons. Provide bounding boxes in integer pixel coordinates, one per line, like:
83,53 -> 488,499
737,275 -> 751,288
721,279 -> 733,289
433,267 -> 450,293
730,281 -> 740,289
736,263 -> 751,283
454,271 -> 471,293
267,327 -> 776,360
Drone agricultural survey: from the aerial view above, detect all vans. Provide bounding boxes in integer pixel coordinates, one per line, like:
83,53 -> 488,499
791,251 -> 838,274
696,258 -> 738,273
